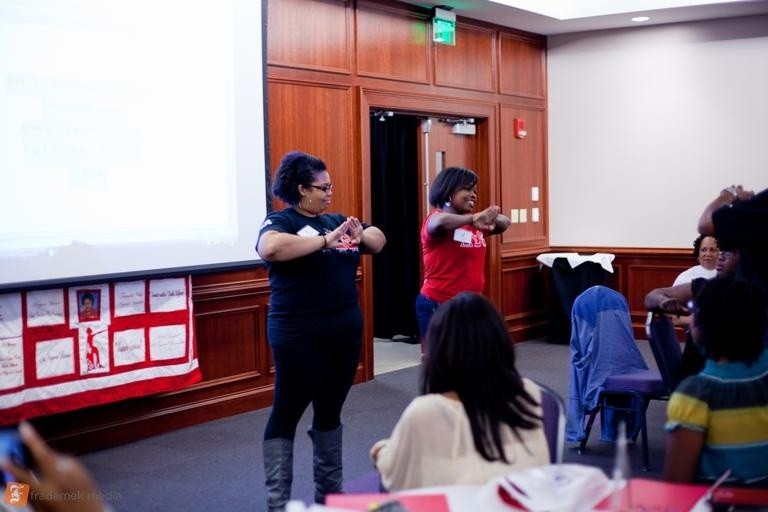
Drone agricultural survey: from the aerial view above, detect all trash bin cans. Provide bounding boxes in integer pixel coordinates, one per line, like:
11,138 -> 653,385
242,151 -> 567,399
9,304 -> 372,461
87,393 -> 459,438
536,253 -> 615,341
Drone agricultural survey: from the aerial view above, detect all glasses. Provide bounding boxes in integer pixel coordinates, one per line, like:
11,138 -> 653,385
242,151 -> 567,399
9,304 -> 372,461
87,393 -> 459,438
309,183 -> 333,194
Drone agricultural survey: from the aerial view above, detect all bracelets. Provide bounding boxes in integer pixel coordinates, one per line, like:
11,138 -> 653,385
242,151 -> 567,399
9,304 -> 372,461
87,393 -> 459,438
323,233 -> 328,249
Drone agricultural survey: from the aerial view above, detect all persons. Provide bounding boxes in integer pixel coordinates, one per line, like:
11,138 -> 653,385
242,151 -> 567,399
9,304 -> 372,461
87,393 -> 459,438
1,423 -> 109,512
645,184 -> 768,512
254,151 -> 386,512
79,294 -> 99,319
85,327 -> 105,371
369,293 -> 570,492
414,166 -> 511,338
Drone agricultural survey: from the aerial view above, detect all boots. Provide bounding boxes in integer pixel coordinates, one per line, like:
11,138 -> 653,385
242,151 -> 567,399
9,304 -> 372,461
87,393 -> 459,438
308,424 -> 343,505
263,438 -> 293,512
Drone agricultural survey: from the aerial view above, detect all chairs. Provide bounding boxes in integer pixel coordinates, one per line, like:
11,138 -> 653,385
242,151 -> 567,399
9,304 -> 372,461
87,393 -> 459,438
530,379 -> 567,464
646,307 -> 683,385
567,284 -> 671,470
